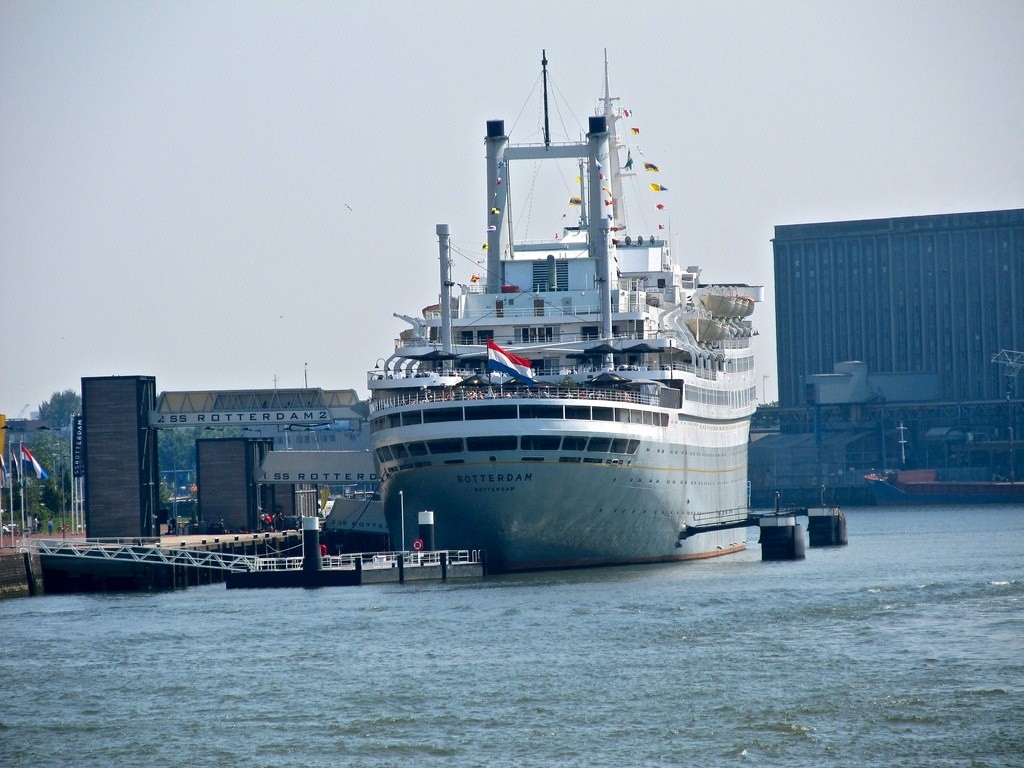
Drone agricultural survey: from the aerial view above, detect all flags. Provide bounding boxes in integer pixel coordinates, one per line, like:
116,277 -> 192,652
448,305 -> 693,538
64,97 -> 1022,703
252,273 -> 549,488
21,444 -> 48,479
488,338 -> 533,385
0,455 -> 6,489
10,449 -> 20,487
470,161 -> 506,284
568,109 -> 668,229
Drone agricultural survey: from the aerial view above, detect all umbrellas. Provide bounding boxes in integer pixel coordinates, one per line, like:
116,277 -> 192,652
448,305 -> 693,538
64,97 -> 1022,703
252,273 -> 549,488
566,342 -> 665,364
584,372 -> 656,385
457,376 -> 494,400
502,376 -> 555,398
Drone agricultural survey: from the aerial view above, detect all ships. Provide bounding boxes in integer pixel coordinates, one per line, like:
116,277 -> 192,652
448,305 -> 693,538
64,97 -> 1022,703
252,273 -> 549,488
366,47 -> 765,573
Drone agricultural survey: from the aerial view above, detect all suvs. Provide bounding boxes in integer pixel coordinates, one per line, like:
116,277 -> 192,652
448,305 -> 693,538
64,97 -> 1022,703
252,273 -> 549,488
283,515 -> 301,530
208,519 -> 229,534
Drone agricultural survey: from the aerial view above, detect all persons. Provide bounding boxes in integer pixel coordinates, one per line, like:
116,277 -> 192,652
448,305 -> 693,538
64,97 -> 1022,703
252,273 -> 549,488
540,390 -> 604,401
409,391 -> 456,404
466,390 -> 511,400
3,524 -> 9,533
260,512 -> 284,532
298,513 -> 302,528
623,392 -> 629,402
48,519 -> 53,535
35,516 -> 42,530
167,517 -> 177,533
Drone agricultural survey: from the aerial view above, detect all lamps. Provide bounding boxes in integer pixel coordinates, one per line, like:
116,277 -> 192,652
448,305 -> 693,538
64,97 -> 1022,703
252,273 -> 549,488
638,235 -> 643,247
204,426 -> 214,431
625,236 -> 631,247
727,296 -> 752,308
303,426 -> 315,435
282,424 -> 294,432
242,426 -> 253,432
714,321 -> 760,339
322,425 -> 331,433
650,235 -> 656,246
696,347 -> 732,364
